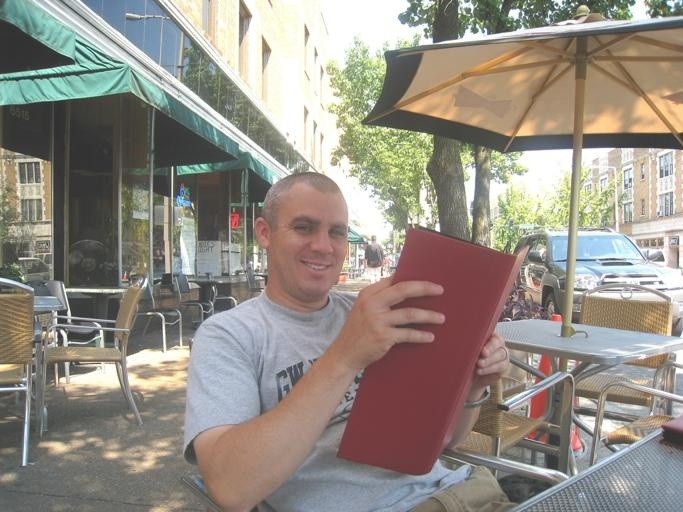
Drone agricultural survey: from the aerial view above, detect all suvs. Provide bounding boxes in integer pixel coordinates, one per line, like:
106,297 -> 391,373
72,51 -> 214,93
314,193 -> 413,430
512,228 -> 683,338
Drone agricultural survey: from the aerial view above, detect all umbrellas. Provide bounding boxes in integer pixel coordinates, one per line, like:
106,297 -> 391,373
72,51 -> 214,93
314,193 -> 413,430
359,5 -> 682,467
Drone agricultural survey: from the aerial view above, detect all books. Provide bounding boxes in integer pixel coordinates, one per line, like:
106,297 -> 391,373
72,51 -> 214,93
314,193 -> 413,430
337,225 -> 528,475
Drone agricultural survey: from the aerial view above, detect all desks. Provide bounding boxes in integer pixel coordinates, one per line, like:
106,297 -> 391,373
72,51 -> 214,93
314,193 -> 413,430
32,296 -> 62,434
510,425 -> 683,511
493,318 -> 680,473
66,284 -> 128,344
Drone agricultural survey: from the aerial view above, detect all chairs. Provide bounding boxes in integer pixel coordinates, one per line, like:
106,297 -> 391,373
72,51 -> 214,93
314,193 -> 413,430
572,283 -> 673,467
461,355 -> 569,458
41,272 -> 148,431
2,273 -> 109,390
129,272 -> 267,353
0,276 -> 34,466
591,359 -> 683,464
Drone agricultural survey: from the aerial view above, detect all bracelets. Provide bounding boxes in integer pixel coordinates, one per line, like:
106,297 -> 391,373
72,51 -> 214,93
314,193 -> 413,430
462,386 -> 492,408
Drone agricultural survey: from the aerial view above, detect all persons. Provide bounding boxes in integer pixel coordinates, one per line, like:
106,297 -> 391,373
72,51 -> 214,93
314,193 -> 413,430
185,173 -> 517,512
365,236 -> 385,283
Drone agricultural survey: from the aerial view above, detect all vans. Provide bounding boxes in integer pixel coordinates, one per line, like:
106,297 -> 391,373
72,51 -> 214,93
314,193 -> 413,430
3,252 -> 53,285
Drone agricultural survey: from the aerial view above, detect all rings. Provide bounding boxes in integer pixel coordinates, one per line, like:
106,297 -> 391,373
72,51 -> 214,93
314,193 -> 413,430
500,346 -> 508,361
